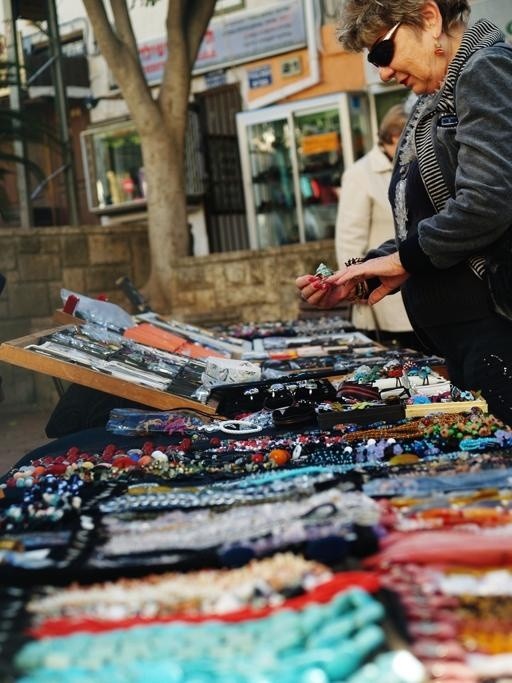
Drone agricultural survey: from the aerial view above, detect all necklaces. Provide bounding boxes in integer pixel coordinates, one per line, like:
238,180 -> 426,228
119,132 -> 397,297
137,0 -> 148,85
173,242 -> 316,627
1,417 -> 512,683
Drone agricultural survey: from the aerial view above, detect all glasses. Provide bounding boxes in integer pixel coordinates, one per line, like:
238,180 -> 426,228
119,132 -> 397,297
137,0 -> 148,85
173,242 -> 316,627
366,19 -> 402,68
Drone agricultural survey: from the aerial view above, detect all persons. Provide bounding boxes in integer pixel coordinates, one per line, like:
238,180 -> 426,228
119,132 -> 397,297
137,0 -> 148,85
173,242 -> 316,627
332,102 -> 416,352
295,1 -> 511,427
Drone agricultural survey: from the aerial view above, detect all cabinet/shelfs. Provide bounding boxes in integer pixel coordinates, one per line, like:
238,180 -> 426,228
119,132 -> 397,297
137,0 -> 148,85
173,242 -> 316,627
79,118 -> 148,214
236,92 -> 364,251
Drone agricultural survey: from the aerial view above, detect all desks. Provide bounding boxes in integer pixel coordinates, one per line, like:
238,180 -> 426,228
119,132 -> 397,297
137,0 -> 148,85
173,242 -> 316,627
0,424 -> 512,682
46,319 -> 511,438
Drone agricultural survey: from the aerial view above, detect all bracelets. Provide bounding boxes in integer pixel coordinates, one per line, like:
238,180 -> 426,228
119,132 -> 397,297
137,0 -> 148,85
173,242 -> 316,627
345,260 -> 368,299
